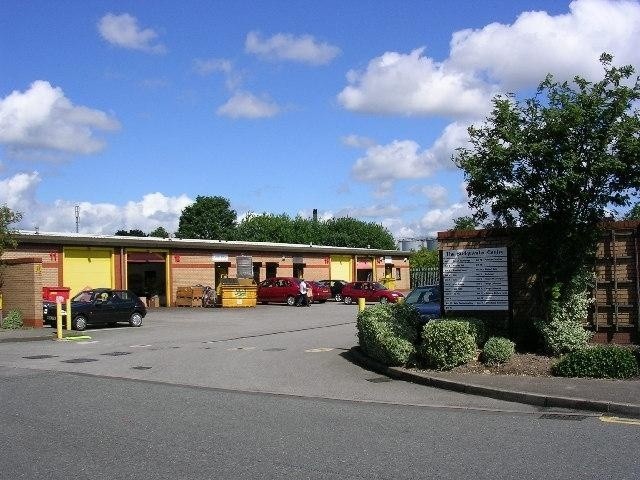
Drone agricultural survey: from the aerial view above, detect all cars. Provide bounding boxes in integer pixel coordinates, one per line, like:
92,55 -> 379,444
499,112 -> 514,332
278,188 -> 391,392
43,288 -> 147,331
405,284 -> 459,319
257,277 -> 405,306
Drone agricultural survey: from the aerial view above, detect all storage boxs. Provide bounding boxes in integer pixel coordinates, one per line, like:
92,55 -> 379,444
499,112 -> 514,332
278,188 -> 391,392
150,298 -> 159,308
